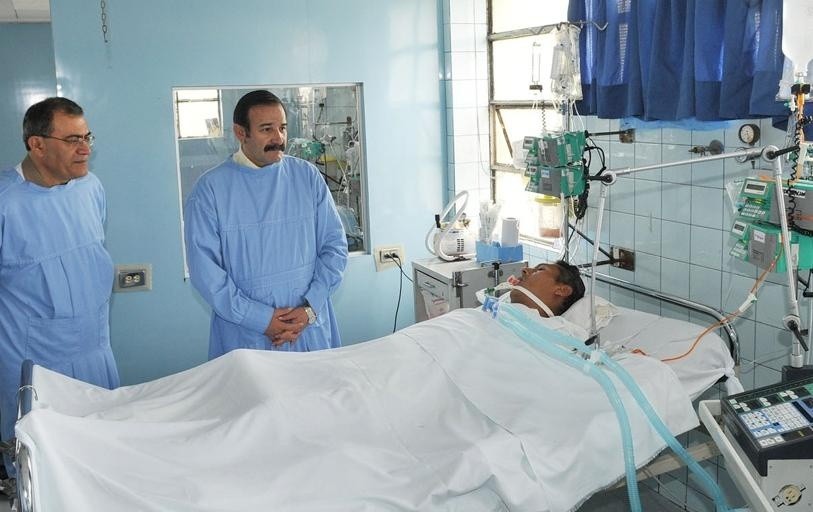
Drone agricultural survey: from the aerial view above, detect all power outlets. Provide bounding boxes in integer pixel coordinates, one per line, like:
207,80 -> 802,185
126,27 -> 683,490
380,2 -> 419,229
372,244 -> 403,273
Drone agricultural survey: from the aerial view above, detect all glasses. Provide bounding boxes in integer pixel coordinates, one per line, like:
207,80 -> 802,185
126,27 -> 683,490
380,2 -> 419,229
41,134 -> 96,148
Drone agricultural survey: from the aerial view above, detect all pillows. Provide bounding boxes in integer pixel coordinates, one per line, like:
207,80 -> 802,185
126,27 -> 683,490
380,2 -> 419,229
559,293 -> 622,334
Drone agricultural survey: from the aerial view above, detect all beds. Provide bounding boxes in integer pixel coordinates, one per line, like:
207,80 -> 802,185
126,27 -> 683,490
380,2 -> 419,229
10,268 -> 741,511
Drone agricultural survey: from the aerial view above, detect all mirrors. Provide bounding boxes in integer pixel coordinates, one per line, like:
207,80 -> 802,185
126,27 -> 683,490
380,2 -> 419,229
171,82 -> 370,280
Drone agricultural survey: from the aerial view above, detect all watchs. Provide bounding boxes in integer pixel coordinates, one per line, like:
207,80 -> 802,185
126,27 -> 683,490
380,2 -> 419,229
303,306 -> 317,325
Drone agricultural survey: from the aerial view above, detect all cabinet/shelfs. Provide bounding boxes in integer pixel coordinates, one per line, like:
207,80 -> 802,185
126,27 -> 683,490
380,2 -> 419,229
411,256 -> 529,325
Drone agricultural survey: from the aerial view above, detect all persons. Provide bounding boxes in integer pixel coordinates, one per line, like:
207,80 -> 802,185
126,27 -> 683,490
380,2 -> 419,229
510,260 -> 585,318
0,97 -> 119,478
183,90 -> 348,363
